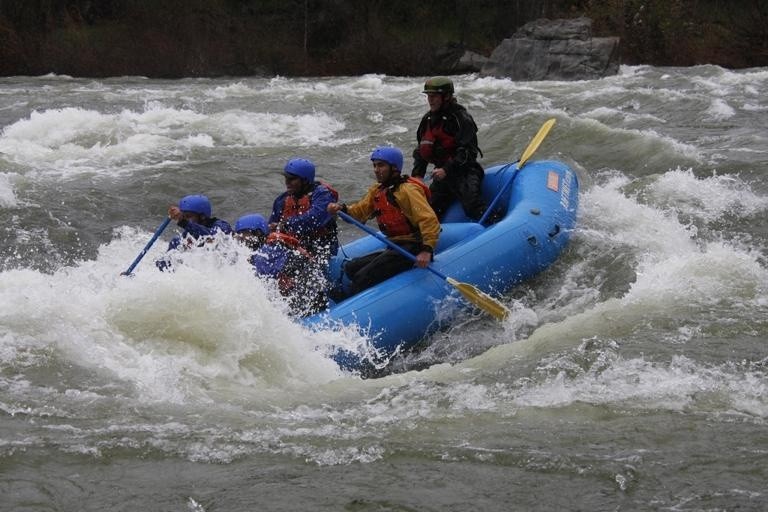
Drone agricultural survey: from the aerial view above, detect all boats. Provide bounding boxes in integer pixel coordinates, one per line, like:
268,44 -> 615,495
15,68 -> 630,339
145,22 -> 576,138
291,162 -> 576,364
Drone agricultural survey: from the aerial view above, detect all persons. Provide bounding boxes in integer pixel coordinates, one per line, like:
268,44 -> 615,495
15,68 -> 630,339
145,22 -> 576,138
160,194 -> 234,255
227,212 -> 331,316
327,148 -> 444,297
265,157 -> 339,255
411,75 -> 490,225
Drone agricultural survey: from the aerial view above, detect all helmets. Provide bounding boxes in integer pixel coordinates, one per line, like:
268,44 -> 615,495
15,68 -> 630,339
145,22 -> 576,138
284,159 -> 314,183
372,147 -> 402,173
180,195 -> 211,218
422,77 -> 454,94
235,214 -> 267,234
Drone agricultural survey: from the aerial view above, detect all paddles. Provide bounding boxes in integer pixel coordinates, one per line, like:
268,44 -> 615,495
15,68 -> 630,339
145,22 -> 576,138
338,209 -> 510,323
479,118 -> 556,225
